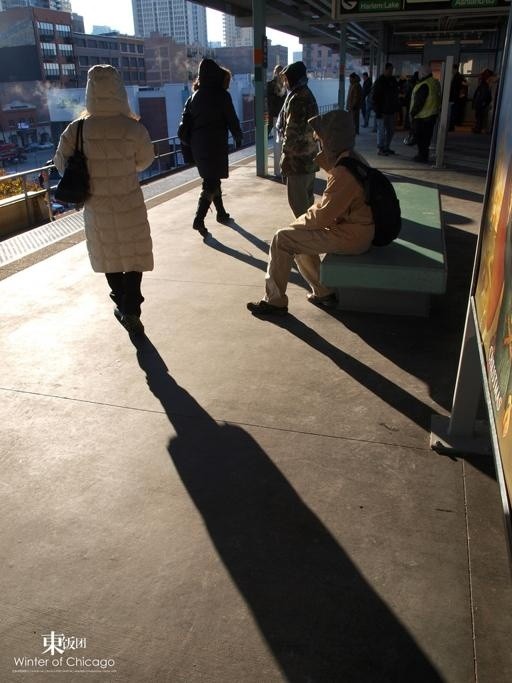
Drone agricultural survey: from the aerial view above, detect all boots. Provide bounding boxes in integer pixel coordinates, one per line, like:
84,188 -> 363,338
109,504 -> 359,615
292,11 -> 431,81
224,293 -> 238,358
213,191 -> 231,224
193,196 -> 210,235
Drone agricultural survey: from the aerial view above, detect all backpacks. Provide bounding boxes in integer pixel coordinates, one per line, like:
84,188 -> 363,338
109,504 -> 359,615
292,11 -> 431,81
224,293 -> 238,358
336,156 -> 401,247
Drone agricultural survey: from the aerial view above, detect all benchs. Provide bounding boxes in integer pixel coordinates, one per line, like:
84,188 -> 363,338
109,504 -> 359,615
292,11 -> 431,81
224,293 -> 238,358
320,178 -> 448,318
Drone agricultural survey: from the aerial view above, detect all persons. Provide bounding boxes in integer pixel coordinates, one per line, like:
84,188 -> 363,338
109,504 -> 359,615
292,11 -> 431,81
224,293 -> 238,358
275,61 -> 320,218
52,63 -> 156,340
177,57 -> 244,239
266,66 -> 287,180
39,169 -> 52,189
247,109 -> 379,318
346,62 -> 502,165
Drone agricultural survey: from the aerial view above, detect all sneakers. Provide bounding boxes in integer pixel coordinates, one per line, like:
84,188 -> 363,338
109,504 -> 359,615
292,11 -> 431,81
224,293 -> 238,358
412,156 -> 426,162
113,306 -> 142,328
378,149 -> 389,155
246,299 -> 288,316
386,147 -> 395,154
307,294 -> 341,307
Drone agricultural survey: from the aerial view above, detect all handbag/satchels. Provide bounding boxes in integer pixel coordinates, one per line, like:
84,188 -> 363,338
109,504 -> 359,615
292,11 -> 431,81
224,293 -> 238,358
401,131 -> 416,146
55,150 -> 90,211
178,141 -> 191,164
